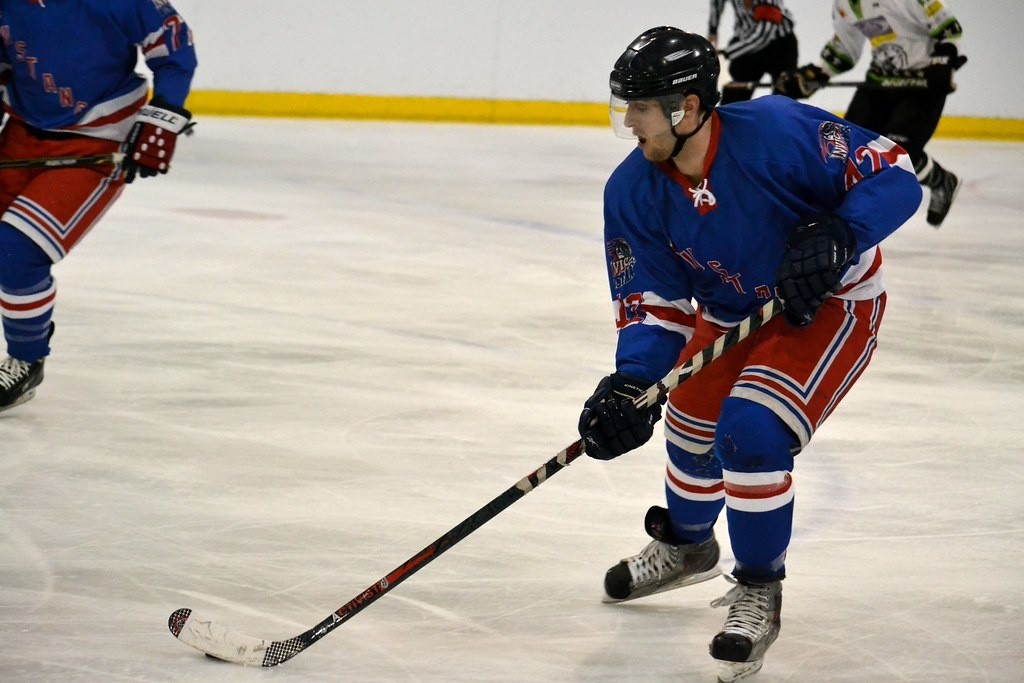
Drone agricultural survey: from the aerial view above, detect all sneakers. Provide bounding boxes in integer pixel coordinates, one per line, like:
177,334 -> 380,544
919,159 -> 960,226
709,574 -> 784,682
0,321 -> 55,414
602,504 -> 724,603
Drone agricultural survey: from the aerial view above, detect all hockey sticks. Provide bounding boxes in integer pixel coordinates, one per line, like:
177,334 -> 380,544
0,149 -> 126,168
718,76 -> 958,88
167,291 -> 786,669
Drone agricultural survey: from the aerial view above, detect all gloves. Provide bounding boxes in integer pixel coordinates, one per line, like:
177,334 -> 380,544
924,41 -> 967,95
777,217 -> 858,328
776,62 -> 830,100
115,96 -> 197,185
577,375 -> 662,461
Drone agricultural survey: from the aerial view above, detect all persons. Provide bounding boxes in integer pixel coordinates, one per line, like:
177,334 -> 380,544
0,0 -> 198,408
579,25 -> 923,664
705,0 -> 963,228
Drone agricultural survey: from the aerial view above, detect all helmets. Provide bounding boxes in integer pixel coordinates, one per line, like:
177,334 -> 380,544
607,25 -> 719,120
